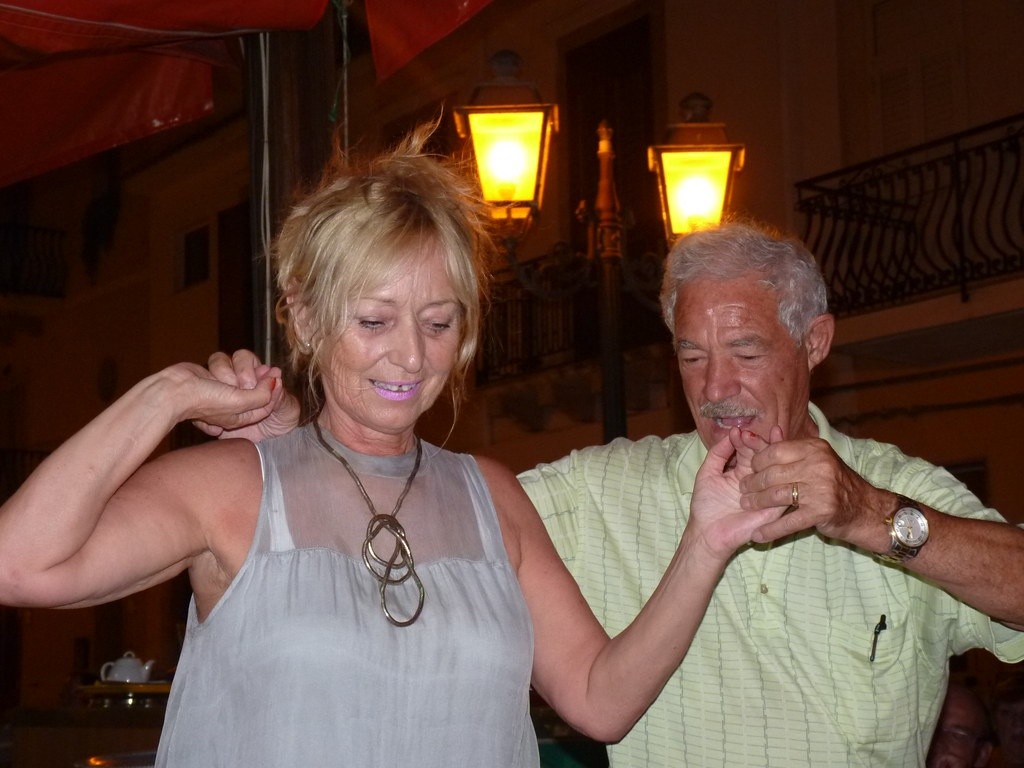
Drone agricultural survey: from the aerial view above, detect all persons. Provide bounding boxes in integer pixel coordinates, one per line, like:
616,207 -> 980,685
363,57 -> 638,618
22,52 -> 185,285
927,657 -> 1024,767
190,221 -> 1024,767
0,154 -> 788,766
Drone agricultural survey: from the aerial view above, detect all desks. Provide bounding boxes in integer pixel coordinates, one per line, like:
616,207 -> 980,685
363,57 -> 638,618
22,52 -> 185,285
79,683 -> 172,711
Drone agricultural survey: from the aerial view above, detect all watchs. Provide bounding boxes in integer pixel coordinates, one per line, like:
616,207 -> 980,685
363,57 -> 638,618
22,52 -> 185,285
869,493 -> 931,567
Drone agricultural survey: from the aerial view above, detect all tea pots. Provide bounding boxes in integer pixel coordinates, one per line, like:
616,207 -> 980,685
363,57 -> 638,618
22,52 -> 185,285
99,650 -> 156,683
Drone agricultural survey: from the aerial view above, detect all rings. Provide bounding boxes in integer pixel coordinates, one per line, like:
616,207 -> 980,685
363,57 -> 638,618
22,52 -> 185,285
790,482 -> 799,507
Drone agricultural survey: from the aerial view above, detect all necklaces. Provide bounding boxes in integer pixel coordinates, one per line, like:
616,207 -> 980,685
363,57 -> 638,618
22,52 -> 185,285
309,415 -> 426,626
